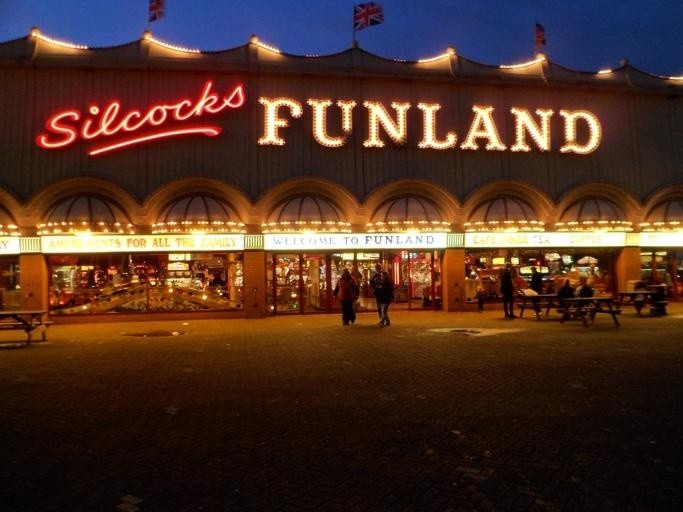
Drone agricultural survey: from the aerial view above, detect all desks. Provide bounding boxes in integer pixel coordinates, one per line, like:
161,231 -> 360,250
0,310 -> 48,345
506,291 -> 670,326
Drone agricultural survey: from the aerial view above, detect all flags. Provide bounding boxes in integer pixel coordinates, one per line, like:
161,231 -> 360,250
353,2 -> 384,30
147,1 -> 165,24
535,21 -> 547,49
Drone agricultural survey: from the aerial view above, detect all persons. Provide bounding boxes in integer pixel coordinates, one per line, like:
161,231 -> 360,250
545,277 -> 555,304
368,262 -> 384,318
332,269 -> 359,327
579,276 -> 592,308
556,279 -> 576,308
500,261 -> 518,319
529,267 -> 540,315
376,269 -> 393,327
572,274 -> 584,296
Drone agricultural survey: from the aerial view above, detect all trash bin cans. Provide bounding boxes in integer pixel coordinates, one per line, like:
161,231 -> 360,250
628,279 -> 646,291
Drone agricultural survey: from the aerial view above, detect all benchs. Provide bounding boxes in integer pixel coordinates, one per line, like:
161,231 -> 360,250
0,321 -> 54,346
